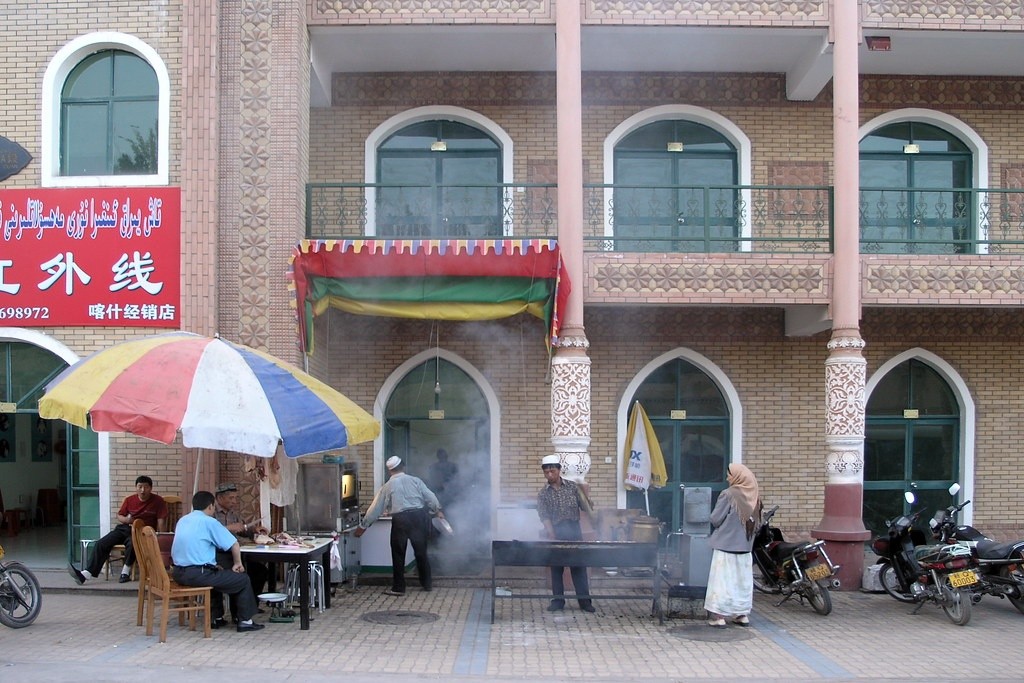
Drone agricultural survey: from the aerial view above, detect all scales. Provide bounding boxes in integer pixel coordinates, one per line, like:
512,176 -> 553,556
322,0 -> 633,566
257,593 -> 295,623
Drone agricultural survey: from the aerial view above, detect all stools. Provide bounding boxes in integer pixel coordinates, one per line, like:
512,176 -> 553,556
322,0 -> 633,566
3,507 -> 33,536
79,539 -> 98,571
287,560 -> 326,615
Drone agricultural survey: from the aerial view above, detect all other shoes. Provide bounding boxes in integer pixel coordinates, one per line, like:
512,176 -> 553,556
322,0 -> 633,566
256,607 -> 265,613
547,600 -> 564,611
732,616 -> 749,627
383,589 -> 404,596
709,619 -> 725,628
580,603 -> 595,612
426,587 -> 432,591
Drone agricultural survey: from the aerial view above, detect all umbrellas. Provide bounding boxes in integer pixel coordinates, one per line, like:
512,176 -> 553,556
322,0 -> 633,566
43,330 -> 380,515
623,401 -> 668,517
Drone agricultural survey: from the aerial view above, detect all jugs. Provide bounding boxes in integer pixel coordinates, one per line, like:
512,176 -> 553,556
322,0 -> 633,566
610,520 -> 630,541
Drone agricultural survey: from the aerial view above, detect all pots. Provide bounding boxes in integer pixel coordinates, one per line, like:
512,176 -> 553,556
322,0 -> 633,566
629,511 -> 661,543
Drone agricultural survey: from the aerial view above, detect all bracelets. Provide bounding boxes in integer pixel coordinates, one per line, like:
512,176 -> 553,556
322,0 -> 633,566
245,524 -> 248,531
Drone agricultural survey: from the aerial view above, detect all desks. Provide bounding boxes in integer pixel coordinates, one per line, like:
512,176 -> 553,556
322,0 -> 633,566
240,537 -> 335,630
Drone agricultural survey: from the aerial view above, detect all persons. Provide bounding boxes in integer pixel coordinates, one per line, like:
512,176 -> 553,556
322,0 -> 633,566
703,462 -> 759,628
172,490 -> 265,632
68,476 -> 169,584
355,449 -> 462,595
536,455 -> 597,612
211,483 -> 270,623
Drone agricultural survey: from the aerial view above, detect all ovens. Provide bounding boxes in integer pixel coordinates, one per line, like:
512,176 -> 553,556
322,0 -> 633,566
304,462 -> 362,583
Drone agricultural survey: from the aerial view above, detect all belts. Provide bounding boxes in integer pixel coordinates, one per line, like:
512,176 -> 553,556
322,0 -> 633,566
404,509 -> 421,513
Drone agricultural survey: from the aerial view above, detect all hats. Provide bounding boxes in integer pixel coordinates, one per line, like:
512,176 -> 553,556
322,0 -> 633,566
215,483 -> 235,492
386,456 -> 401,470
542,454 -> 559,464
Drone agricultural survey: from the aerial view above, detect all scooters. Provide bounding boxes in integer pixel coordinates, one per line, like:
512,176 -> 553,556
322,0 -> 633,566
872,492 -> 983,625
928,482 -> 1024,615
753,505 -> 841,616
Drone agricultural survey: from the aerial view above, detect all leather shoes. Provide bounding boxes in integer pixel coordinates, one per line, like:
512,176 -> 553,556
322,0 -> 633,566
67,562 -> 85,585
119,572 -> 130,583
237,620 -> 265,632
211,618 -> 228,629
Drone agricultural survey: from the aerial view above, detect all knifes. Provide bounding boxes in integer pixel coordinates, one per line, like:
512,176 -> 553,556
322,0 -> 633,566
235,515 -> 266,533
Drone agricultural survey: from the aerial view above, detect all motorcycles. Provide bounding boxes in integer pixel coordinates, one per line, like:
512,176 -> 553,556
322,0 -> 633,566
0,545 -> 42,628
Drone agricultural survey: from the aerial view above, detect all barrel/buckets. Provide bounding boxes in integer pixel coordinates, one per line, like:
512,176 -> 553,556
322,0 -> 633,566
685,489 -> 709,522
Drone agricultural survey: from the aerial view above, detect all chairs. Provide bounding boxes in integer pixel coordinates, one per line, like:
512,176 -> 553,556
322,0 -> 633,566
141,526 -> 213,642
163,495 -> 183,536
104,544 -> 138,581
131,518 -> 184,626
37,488 -> 59,536
